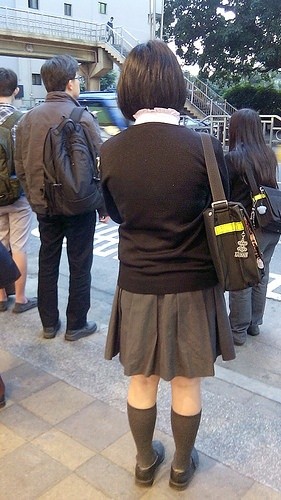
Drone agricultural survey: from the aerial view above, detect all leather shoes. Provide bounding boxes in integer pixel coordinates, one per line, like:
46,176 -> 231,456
43,319 -> 61,338
65,320 -> 97,341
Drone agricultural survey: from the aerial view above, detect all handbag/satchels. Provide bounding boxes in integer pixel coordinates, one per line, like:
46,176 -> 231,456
236,144 -> 280,234
201,132 -> 265,290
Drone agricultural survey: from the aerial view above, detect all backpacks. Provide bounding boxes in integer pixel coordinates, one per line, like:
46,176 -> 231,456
0,110 -> 25,206
39,106 -> 104,221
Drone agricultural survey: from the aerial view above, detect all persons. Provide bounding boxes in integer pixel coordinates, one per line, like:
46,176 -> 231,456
14,54 -> 111,343
100,38 -> 232,493
0,66 -> 41,314
214,107 -> 281,345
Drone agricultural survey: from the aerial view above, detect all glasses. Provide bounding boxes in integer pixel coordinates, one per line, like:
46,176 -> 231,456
66,75 -> 82,82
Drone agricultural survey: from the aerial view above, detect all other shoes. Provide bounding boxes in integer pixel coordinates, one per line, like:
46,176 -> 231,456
0,298 -> 14,312
0,377 -> 6,408
135,440 -> 164,486
12,296 -> 38,313
247,324 -> 260,336
169,446 -> 199,490
233,337 -> 246,346
